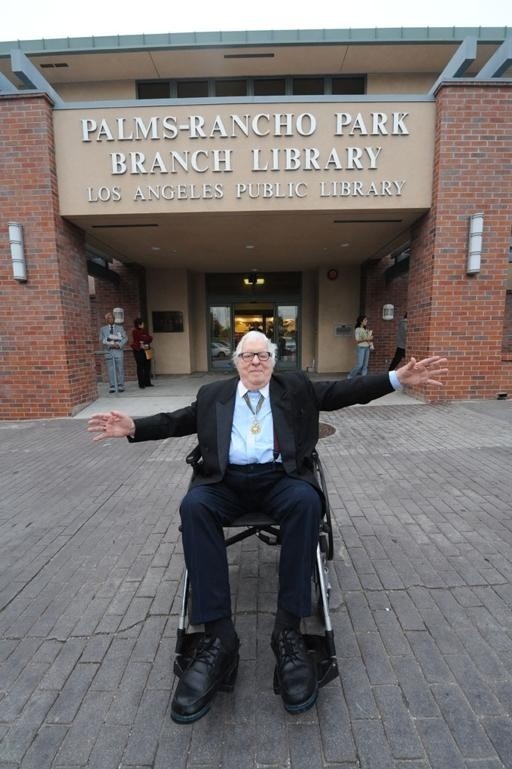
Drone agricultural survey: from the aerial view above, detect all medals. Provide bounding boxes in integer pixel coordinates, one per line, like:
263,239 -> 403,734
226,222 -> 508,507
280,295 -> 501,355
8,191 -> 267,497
238,391 -> 268,435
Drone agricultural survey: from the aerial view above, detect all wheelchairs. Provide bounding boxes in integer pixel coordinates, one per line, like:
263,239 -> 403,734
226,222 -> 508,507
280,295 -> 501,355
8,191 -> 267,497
172,439 -> 341,698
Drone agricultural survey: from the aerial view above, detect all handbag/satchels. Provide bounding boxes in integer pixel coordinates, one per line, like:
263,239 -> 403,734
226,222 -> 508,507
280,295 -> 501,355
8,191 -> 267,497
144,349 -> 154,360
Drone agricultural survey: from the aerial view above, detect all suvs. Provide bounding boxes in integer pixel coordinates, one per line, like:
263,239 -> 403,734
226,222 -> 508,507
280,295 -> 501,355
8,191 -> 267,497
210,341 -> 231,360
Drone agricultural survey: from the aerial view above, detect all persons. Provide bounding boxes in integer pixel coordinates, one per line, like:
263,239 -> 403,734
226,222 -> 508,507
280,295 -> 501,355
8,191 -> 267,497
346,316 -> 374,380
98,312 -> 129,393
388,312 -> 408,370
131,318 -> 154,389
87,329 -> 451,723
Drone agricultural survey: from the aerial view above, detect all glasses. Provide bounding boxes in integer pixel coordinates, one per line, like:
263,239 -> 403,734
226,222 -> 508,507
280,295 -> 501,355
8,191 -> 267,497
237,351 -> 274,363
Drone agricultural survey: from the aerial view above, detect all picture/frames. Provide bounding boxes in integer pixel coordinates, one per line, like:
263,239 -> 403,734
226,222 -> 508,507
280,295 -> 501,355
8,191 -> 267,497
328,269 -> 338,280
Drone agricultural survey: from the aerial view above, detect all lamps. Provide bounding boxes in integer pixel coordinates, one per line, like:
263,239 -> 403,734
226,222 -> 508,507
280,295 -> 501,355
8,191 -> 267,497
238,272 -> 266,303
112,308 -> 125,324
7,222 -> 26,281
382,304 -> 395,320
466,212 -> 484,275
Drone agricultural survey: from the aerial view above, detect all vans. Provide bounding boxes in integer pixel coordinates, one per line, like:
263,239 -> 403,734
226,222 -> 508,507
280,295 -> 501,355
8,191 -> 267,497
280,335 -> 297,354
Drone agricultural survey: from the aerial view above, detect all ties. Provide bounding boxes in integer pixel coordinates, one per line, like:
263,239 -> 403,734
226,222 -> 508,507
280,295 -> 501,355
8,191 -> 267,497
108,325 -> 114,336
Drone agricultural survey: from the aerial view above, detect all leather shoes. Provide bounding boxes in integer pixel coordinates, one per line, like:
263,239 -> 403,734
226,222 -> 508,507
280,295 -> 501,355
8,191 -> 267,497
271,628 -> 321,716
167,633 -> 240,726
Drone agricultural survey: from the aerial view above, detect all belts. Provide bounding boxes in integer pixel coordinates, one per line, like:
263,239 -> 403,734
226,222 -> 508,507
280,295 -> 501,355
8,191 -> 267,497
226,462 -> 283,473
